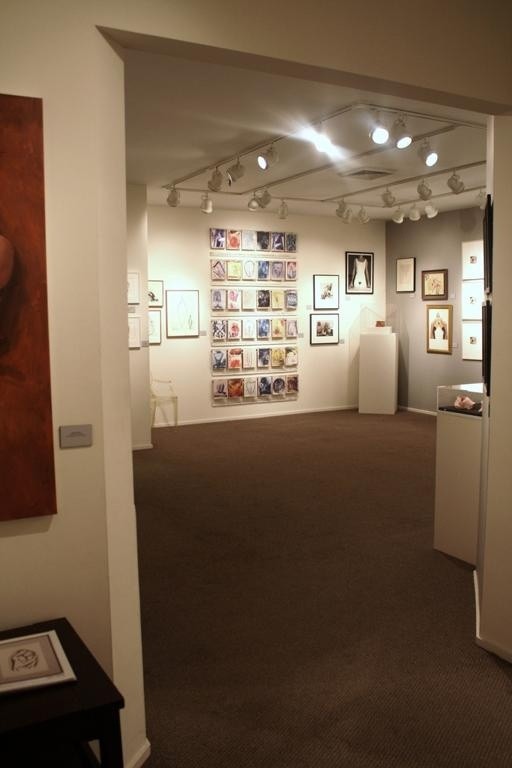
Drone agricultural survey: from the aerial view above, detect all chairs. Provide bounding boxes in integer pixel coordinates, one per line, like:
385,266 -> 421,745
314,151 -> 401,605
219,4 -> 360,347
148,361 -> 178,432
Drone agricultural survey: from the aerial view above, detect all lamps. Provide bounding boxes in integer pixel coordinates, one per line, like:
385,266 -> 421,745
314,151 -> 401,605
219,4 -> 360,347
247,188 -> 270,212
336,202 -> 348,217
367,111 -> 391,145
418,137 -> 439,167
342,209 -> 353,224
424,202 -> 439,218
409,207 -> 420,221
448,172 -> 465,194
259,145 -> 279,170
165,185 -> 181,208
200,195 -> 212,214
392,210 -> 404,224
416,178 -> 433,201
358,209 -> 370,223
389,115 -> 414,148
381,185 -> 397,208
279,200 -> 288,220
227,158 -> 245,183
208,168 -> 223,192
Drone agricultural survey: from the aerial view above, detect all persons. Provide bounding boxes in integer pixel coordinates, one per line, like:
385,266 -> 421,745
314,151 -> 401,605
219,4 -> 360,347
348,255 -> 371,291
214,232 -> 225,248
317,322 -> 333,337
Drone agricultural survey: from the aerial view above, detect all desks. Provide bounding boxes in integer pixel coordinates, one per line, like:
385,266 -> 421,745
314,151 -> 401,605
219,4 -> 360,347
432,382 -> 484,566
0,617 -> 124,767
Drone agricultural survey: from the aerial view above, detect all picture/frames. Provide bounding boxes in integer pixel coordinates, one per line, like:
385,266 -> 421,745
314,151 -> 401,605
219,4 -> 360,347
128,314 -> 142,350
310,313 -> 340,344
0,630 -> 77,692
166,290 -> 201,337
345,250 -> 374,294
126,272 -> 139,304
147,279 -> 163,307
394,256 -> 453,353
209,227 -> 299,398
461,239 -> 484,361
149,310 -> 162,345
313,274 -> 339,311
